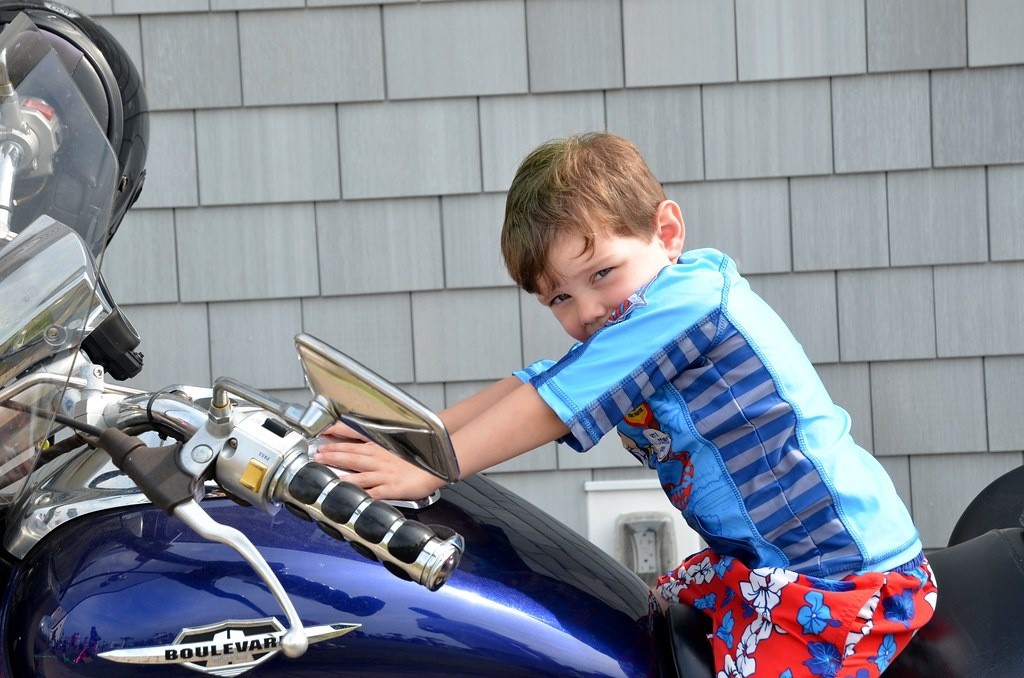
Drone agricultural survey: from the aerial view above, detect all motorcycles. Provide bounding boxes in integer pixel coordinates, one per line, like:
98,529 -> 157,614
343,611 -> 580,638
0,9 -> 1024,678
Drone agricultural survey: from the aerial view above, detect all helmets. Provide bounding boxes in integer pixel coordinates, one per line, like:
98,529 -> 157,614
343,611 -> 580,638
0,0 -> 151,257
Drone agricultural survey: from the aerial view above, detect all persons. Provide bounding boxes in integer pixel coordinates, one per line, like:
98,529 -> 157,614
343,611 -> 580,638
312,133 -> 937,678
